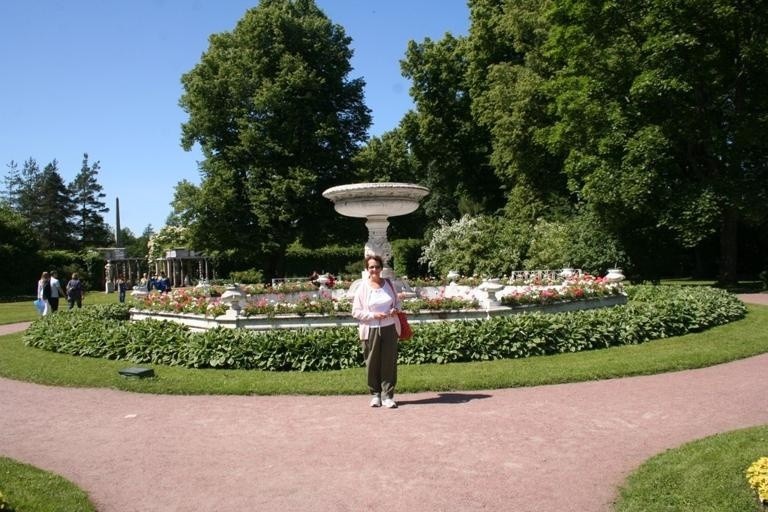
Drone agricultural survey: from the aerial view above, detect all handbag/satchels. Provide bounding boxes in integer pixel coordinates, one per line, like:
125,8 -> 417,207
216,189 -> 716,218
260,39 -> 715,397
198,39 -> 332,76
67,287 -> 77,297
397,312 -> 412,338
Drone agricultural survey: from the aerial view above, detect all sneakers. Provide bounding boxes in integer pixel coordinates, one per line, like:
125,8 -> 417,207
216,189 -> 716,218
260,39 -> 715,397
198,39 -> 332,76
371,398 -> 381,407
382,397 -> 396,408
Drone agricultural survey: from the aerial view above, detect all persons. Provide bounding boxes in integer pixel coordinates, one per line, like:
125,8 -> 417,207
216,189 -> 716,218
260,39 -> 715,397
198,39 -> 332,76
311,270 -> 320,288
325,272 -> 335,288
48,270 -> 65,314
352,255 -> 402,409
115,270 -> 172,303
36,271 -> 49,317
65,272 -> 85,309
